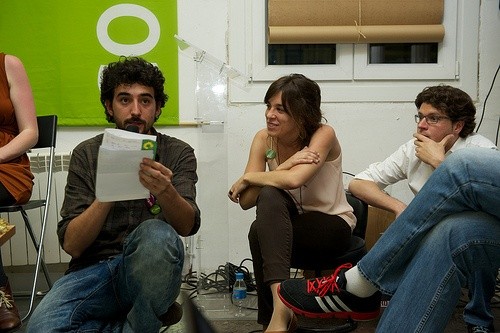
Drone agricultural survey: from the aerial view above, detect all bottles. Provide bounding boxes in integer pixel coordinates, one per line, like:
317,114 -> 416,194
233,273 -> 248,317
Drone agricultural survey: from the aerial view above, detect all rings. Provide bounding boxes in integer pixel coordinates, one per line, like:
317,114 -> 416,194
228,190 -> 233,195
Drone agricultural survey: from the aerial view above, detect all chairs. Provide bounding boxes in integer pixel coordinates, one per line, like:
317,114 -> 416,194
285,170 -> 368,333
0,114 -> 59,323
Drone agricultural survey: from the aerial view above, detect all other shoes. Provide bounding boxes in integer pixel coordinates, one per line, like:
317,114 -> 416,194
158,301 -> 185,333
264,313 -> 298,333
0,277 -> 21,331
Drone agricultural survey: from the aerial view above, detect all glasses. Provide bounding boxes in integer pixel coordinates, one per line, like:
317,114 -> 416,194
414,114 -> 451,126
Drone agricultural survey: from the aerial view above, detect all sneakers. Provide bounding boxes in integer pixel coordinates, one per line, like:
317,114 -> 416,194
277,263 -> 388,321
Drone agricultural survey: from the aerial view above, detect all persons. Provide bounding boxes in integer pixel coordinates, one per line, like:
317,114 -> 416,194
349,83 -> 500,220
227,74 -> 357,333
277,143 -> 500,333
0,53 -> 39,333
24,55 -> 201,333
